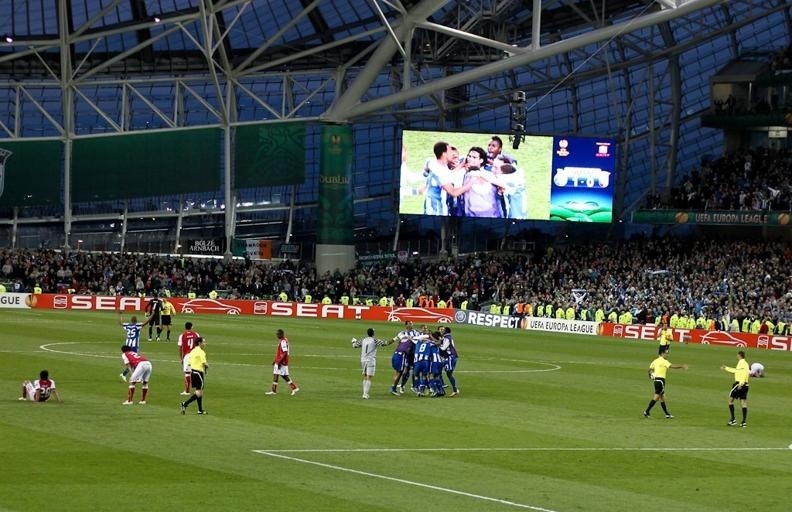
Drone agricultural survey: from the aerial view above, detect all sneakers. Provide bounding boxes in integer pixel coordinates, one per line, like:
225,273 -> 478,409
390,383 -> 459,396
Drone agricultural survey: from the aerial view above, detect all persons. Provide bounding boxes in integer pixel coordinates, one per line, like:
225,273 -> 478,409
707,91 -> 792,117
399,135 -> 529,219
644,346 -> 688,418
178,322 -> 208,414
391,321 -> 459,397
658,324 -> 673,355
351,328 -> 392,399
121,345 -> 153,404
0,229 -> 791,336
721,351 -> 751,426
19,370 -> 62,402
120,308 -> 158,382
645,141 -> 792,214
265,329 -> 299,395
